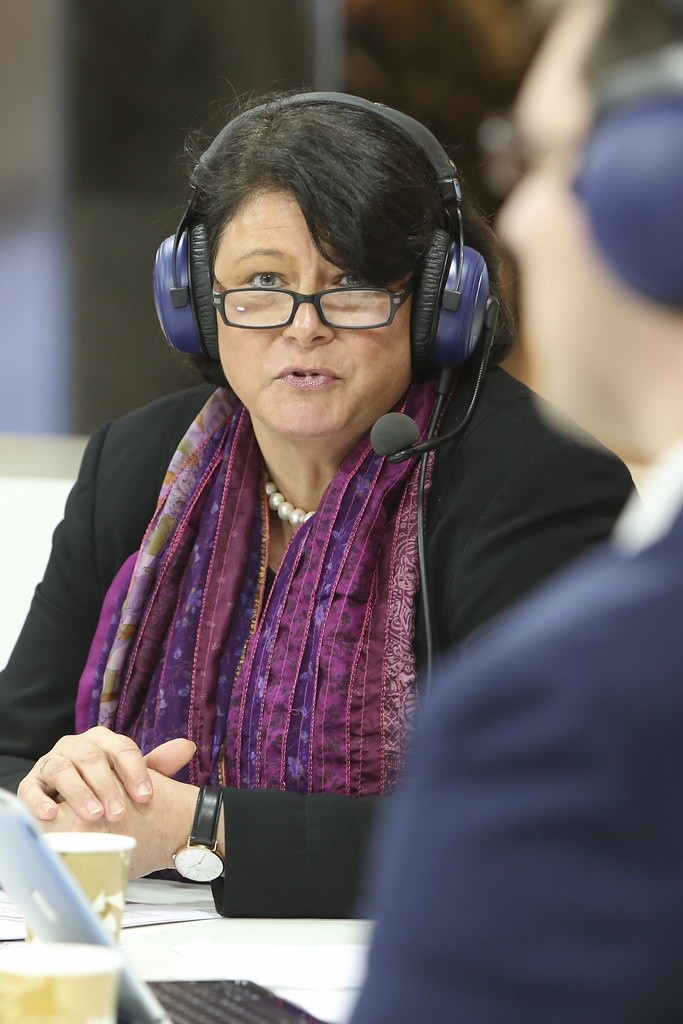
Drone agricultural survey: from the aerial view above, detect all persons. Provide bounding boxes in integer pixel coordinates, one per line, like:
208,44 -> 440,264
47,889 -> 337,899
0,0 -> 683,1024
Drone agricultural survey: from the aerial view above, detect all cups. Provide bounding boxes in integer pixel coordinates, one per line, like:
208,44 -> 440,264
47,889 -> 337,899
45,832 -> 136,941
0,941 -> 120,1024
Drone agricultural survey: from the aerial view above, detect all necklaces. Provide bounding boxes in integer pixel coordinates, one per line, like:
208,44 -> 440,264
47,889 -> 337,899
262,473 -> 319,524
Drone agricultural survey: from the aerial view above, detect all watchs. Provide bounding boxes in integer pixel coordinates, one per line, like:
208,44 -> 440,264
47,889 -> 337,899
170,781 -> 225,883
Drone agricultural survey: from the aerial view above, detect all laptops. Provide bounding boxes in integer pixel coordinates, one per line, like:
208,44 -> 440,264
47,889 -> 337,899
0,786 -> 331,1024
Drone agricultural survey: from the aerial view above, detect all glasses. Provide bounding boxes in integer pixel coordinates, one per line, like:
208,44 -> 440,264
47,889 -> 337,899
210,276 -> 414,329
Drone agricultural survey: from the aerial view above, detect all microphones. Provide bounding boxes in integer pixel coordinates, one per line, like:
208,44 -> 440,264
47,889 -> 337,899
371,347 -> 492,464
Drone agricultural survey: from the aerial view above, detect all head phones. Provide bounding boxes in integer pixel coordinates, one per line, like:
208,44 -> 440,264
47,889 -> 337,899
569,0 -> 683,315
150,91 -> 500,386
